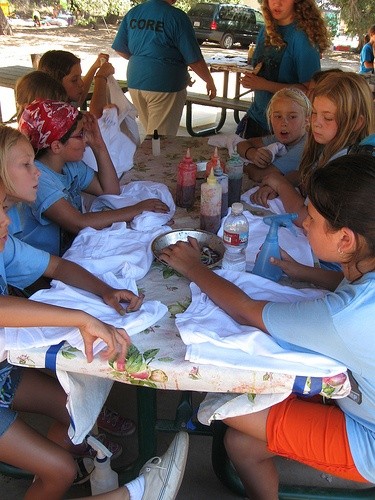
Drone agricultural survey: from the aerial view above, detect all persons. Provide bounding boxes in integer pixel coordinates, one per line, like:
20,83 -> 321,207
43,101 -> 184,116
0,71 -> 120,172
236,88 -> 314,182
8,2 -> 61,27
250,70 -> 374,231
358,25 -> 374,74
157,148 -> 375,500
66,432 -> 189,500
110,1 -> 218,137
0,123 -> 138,461
38,49 -> 115,121
12,97 -> 171,295
232,0 -> 335,139
0,176 -> 145,500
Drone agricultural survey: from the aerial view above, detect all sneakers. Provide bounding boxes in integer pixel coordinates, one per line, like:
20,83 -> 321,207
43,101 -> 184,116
96,405 -> 136,436
71,456 -> 95,485
138,431 -> 189,499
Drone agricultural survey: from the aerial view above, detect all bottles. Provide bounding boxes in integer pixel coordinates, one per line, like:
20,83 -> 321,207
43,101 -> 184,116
83,436 -> 118,497
200,146 -> 244,236
220,203 -> 248,274
173,149 -> 197,208
248,44 -> 255,65
150,130 -> 161,157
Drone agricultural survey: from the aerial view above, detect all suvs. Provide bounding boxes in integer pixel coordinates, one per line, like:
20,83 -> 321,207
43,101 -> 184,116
184,2 -> 267,49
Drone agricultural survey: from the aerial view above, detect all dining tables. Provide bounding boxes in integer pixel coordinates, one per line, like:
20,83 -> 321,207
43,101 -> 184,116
6,132 -> 375,499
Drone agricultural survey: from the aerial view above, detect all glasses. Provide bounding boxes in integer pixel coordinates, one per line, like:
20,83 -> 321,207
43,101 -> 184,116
68,127 -> 88,139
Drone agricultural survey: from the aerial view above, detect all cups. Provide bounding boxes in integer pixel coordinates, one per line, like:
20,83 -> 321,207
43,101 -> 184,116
31,53 -> 41,69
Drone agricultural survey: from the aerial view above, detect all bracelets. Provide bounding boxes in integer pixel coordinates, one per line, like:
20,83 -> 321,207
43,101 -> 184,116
244,146 -> 253,160
94,75 -> 107,83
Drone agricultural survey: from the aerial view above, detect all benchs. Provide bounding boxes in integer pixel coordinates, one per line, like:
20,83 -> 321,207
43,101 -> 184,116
183,52 -> 255,137
0,64 -> 129,127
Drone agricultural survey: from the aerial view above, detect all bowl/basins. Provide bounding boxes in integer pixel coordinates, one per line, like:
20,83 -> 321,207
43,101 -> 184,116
151,228 -> 223,269
